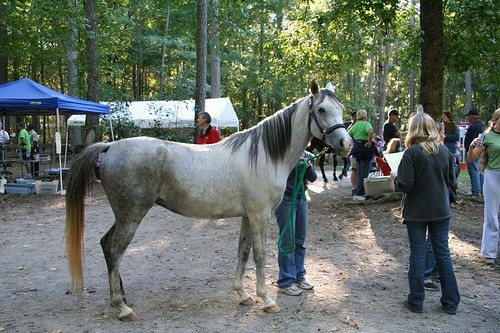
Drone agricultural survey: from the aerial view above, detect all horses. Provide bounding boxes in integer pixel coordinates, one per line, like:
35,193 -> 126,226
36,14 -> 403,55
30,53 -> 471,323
61,76 -> 355,322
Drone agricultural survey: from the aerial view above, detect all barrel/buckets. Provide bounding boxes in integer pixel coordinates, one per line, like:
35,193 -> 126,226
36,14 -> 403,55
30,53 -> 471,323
459,162 -> 467,170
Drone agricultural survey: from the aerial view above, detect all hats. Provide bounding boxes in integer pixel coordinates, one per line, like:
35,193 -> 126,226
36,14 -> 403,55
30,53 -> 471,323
463,107 -> 480,115
388,109 -> 401,116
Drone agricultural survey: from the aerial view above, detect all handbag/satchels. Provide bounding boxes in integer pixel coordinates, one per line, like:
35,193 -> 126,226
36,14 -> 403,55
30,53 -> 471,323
351,139 -> 370,159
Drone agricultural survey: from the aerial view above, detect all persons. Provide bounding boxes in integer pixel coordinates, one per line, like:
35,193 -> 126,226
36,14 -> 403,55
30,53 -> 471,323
195,110 -> 221,147
27,123 -> 40,177
418,121 -> 460,291
16,122 -> 32,178
337,105 -> 487,210
0,122 -> 10,167
92,150 -> 105,183
275,146 -> 317,295
466,106 -> 500,268
393,111 -> 463,314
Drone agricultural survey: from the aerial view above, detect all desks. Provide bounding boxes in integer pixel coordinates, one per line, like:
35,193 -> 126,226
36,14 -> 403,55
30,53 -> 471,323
0,157 -> 51,183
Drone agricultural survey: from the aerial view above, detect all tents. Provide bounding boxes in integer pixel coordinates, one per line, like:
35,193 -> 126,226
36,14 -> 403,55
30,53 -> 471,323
63,97 -> 235,169
0,76 -> 115,196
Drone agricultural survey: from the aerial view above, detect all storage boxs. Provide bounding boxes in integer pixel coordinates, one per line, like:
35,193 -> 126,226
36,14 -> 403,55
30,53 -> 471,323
3,183 -> 35,195
35,179 -> 59,195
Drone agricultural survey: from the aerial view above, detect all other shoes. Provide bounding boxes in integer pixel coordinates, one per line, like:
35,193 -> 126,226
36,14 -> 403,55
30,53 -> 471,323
423,279 -> 440,292
293,275 -> 314,289
484,258 -> 496,266
278,280 -> 303,297
351,192 -> 366,201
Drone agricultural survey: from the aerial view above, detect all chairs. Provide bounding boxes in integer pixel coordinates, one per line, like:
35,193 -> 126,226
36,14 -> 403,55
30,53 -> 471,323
364,157 -> 398,185
